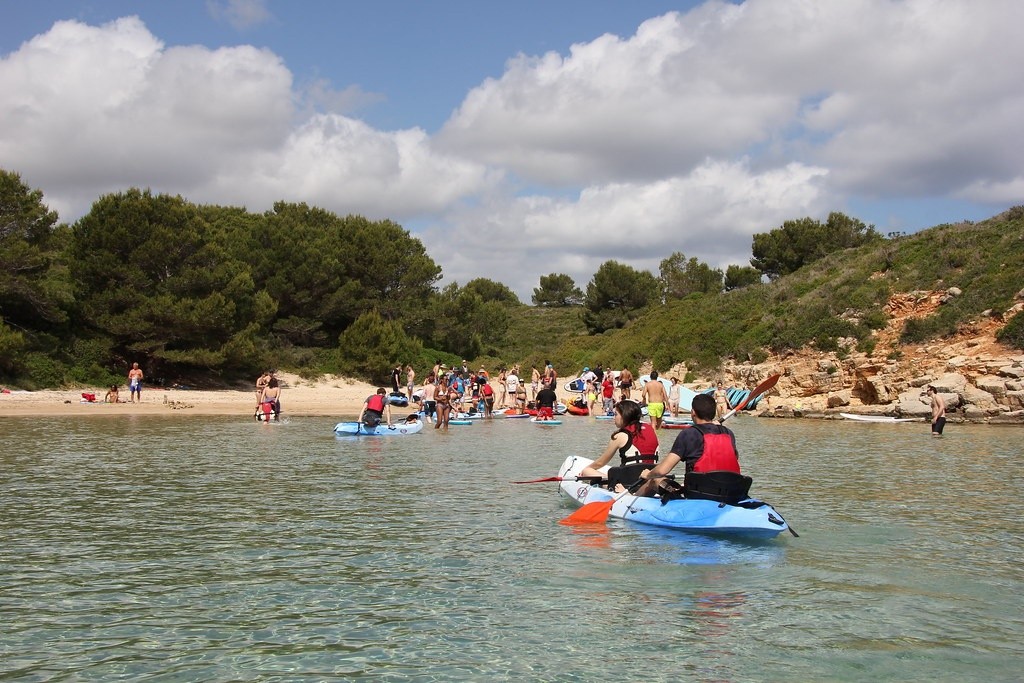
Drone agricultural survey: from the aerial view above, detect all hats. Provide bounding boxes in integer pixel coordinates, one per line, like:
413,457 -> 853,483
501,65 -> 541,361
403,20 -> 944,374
481,364 -> 484,368
478,369 -> 486,372
582,367 -> 589,371
440,364 -> 447,369
462,360 -> 467,363
546,365 -> 553,368
519,379 -> 524,382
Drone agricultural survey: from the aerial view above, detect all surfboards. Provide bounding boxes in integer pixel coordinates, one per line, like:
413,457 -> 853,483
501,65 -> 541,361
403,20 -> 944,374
839,413 -> 911,422
433,417 -> 472,425
595,415 -> 613,419
639,374 -> 700,412
532,416 -> 563,425
505,414 -> 530,418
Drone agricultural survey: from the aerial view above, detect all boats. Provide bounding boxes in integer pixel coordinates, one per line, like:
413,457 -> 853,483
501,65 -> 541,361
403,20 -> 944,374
552,401 -> 568,415
387,393 -> 408,407
333,418 -> 424,438
556,455 -> 790,541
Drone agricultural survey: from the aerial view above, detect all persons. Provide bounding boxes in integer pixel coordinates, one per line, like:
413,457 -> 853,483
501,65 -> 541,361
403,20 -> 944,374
581,400 -> 660,495
406,365 -> 415,402
414,359 -> 558,429
927,385 -> 946,435
358,388 -> 395,427
105,384 -> 126,403
391,364 -> 402,393
615,394 -> 740,505
128,362 -> 143,404
714,382 -> 732,419
254,369 -> 281,422
580,362 -> 680,430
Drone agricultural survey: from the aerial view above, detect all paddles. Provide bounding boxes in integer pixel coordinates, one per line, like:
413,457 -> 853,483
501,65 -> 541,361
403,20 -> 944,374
511,473 -> 685,485
557,373 -> 780,524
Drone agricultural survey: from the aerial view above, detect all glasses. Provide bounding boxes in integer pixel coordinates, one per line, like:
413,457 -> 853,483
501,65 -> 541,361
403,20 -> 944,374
518,381 -> 524,383
444,379 -> 449,381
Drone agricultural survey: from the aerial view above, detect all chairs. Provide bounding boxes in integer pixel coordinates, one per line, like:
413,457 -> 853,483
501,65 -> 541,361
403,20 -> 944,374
683,471 -> 753,505
608,463 -> 658,496
